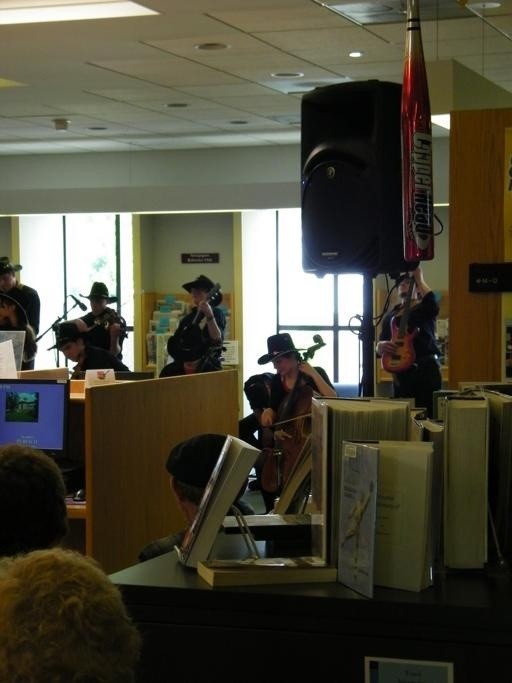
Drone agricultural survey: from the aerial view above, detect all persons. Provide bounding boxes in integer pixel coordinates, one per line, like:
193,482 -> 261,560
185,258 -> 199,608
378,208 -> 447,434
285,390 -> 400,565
61,282 -> 128,361
49,324 -> 127,377
238,372 -> 275,491
375,265 -> 442,419
0,256 -> 40,334
178,275 -> 226,346
159,327 -> 222,377
254,334 -> 338,511
1,445 -> 140,683
0,286 -> 37,370
138,433 -> 255,561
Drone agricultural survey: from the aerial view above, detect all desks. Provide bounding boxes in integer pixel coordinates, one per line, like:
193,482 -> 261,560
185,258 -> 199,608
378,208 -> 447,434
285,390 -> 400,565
104,533 -> 510,683
0,368 -> 240,575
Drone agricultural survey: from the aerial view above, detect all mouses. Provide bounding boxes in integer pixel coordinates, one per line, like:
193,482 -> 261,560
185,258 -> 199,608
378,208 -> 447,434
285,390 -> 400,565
73,485 -> 86,506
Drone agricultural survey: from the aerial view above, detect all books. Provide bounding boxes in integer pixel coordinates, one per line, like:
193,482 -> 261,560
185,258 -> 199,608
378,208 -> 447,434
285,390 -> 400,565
174,435 -> 337,588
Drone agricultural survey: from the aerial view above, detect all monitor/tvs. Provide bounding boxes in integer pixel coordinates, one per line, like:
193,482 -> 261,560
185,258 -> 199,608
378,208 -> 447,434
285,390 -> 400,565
0,378 -> 70,458
74,370 -> 155,381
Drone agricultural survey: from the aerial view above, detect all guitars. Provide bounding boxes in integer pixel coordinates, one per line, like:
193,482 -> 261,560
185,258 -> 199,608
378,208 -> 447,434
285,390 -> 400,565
382,275 -> 418,372
167,282 -> 220,360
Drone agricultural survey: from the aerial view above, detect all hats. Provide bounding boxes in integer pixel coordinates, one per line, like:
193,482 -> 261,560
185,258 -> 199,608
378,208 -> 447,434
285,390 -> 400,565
79,282 -> 118,304
182,275 -> 223,308
258,333 -> 306,364
165,433 -> 249,496
1,257 -> 22,275
46,320 -> 98,352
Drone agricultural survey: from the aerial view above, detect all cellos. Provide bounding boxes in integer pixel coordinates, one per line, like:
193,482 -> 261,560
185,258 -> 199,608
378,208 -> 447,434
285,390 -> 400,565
261,335 -> 326,493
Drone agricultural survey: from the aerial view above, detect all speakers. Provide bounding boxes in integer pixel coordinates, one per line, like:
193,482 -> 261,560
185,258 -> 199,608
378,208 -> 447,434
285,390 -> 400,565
300,80 -> 421,275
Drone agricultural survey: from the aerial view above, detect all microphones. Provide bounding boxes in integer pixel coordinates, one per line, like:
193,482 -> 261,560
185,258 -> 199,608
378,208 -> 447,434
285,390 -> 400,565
69,294 -> 87,312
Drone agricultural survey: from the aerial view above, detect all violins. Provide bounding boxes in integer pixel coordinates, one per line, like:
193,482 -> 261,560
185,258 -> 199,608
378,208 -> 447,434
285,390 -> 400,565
94,309 -> 129,339
